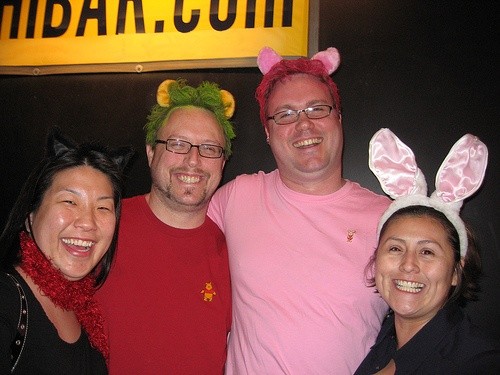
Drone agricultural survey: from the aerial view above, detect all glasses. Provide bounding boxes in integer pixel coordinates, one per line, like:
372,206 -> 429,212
266,105 -> 335,125
154,138 -> 225,160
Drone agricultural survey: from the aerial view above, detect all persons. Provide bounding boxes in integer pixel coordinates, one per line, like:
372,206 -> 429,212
0,147 -> 122,375
354,206 -> 500,375
94,78 -> 237,375
208,56 -> 394,375
